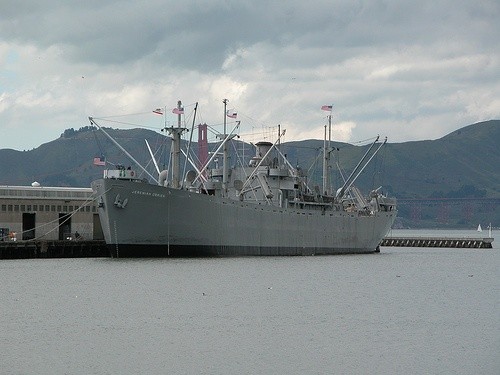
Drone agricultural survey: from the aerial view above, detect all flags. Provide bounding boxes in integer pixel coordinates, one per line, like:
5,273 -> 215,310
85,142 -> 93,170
321,105 -> 332,111
93,156 -> 106,166
226,110 -> 237,118
153,108 -> 166,115
172,106 -> 185,115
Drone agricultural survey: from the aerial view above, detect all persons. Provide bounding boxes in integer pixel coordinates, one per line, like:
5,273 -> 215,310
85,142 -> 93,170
75,231 -> 80,239
0,229 -> 18,242
66,235 -> 72,240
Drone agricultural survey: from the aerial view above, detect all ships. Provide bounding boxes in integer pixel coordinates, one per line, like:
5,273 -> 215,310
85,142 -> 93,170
89,100 -> 400,258
